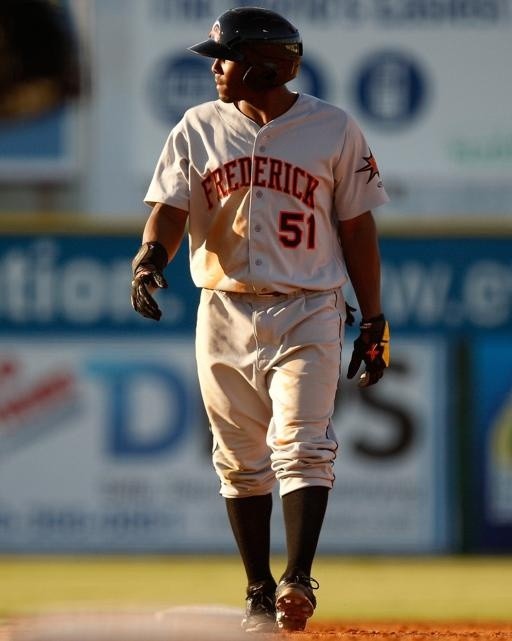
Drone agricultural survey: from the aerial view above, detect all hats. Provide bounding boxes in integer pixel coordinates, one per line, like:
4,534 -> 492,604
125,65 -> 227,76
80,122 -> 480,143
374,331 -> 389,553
186,6 -> 304,90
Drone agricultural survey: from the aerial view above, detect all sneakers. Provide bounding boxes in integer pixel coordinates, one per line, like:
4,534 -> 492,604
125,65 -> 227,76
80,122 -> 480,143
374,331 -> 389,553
274,574 -> 318,632
240,580 -> 279,634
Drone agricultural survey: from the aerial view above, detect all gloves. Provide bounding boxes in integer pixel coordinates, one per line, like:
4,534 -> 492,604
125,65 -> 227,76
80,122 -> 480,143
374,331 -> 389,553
128,239 -> 170,321
345,313 -> 393,389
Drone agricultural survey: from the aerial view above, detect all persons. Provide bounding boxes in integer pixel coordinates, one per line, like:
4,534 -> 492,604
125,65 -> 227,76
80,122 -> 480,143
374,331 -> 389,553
0,0 -> 90,214
127,4 -> 392,630
